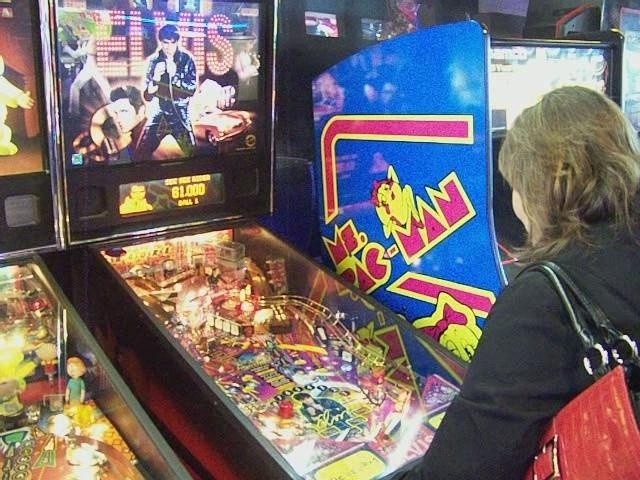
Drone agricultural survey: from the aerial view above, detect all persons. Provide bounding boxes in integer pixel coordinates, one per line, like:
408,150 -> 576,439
108,84 -> 153,162
200,49 -> 243,111
230,30 -> 261,84
61,26 -> 118,112
0,53 -> 36,159
378,82 -> 640,480
125,24 -> 200,163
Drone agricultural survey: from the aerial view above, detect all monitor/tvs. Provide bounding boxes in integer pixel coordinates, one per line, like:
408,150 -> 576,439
491,37 -> 624,141
360,18 -> 389,41
304,10 -> 339,38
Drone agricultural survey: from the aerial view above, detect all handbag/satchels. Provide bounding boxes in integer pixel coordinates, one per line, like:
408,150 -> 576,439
523,364 -> 640,480
